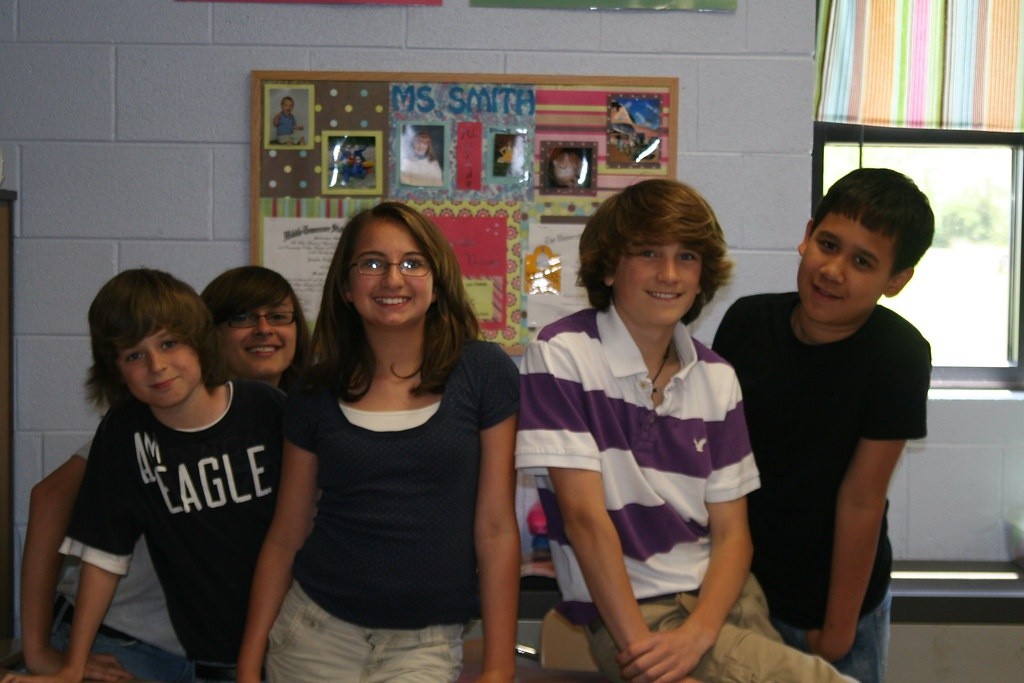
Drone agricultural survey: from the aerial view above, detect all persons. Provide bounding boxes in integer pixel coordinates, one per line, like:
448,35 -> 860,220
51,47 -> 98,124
0,265 -> 290,683
708,165 -> 936,683
272,95 -> 305,145
496,137 -> 522,172
236,199 -> 523,683
401,130 -> 443,186
511,179 -> 868,683
15,262 -> 311,683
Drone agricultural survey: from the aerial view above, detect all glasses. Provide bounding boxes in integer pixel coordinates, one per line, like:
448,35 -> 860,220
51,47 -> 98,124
349,258 -> 435,276
227,310 -> 297,328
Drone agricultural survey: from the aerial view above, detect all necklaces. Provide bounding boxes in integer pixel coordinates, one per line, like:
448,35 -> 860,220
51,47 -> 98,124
650,341 -> 676,396
